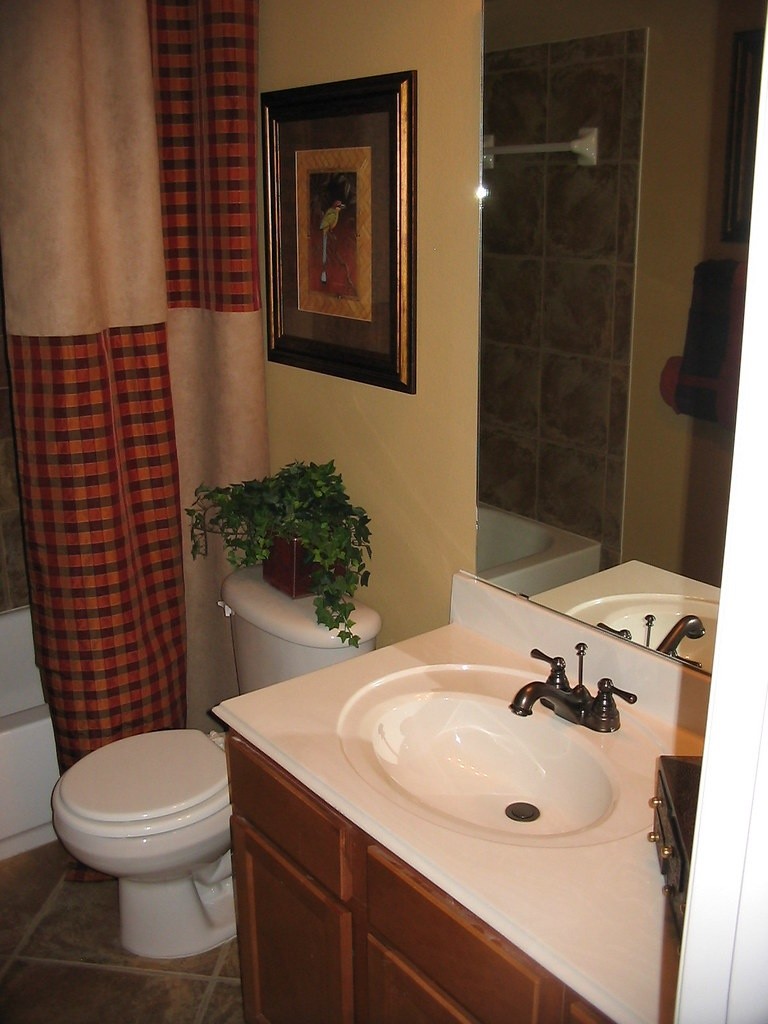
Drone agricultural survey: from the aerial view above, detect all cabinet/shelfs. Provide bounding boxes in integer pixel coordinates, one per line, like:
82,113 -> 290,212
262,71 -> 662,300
226,727 -> 616,1024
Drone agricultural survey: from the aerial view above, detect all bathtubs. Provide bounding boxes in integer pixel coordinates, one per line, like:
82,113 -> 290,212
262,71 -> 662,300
474,496 -> 604,597
0,604 -> 63,864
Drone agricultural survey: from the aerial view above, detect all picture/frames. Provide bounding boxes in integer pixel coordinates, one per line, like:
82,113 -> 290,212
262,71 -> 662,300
260,70 -> 418,396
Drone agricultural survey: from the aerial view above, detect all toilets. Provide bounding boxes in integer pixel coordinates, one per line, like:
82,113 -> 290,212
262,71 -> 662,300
52,564 -> 381,963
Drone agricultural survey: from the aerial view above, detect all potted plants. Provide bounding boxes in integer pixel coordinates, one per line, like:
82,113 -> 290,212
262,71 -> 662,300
184,459 -> 371,649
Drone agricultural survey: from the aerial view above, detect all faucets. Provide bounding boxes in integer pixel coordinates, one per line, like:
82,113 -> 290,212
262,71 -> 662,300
562,593 -> 720,672
508,641 -> 637,732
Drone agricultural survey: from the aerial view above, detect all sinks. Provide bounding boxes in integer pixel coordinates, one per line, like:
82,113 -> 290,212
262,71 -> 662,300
337,665 -> 665,849
603,604 -> 717,679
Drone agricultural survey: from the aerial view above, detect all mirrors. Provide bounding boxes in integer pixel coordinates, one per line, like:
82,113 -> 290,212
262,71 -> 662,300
475,0 -> 768,679
